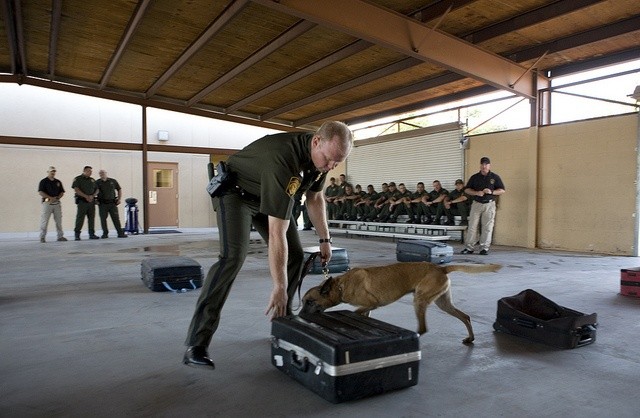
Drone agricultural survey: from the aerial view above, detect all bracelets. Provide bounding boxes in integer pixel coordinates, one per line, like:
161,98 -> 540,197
492,190 -> 494,195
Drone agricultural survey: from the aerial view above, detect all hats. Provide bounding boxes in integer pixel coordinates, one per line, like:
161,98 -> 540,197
388,181 -> 395,186
455,179 -> 463,184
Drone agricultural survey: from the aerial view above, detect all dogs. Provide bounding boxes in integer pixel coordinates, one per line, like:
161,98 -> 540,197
299,262 -> 503,344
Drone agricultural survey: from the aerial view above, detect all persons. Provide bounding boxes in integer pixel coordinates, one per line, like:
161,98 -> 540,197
443,180 -> 473,225
404,182 -> 429,224
379,184 -> 411,223
334,185 -> 354,220
324,177 -> 338,228
373,182 -> 401,222
333,173 -> 349,221
353,185 -> 378,221
462,157 -> 506,255
360,183 -> 388,222
96,168 -> 128,238
342,185 -> 366,222
72,166 -> 99,240
38,165 -> 68,242
422,180 -> 448,224
182,121 -> 355,369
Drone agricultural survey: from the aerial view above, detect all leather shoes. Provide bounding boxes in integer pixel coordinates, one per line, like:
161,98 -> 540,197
118,233 -> 127,237
75,235 -> 80,239
459,220 -> 467,225
406,217 -> 433,223
183,344 -> 214,368
102,233 -> 108,237
57,237 -> 67,240
480,249 -> 487,254
432,220 -> 440,224
357,215 -> 396,222
337,213 -> 356,220
89,234 -> 99,238
461,248 -> 473,253
41,238 -> 45,242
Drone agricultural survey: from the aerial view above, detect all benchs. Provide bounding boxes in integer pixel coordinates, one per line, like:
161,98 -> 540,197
328,219 -> 467,233
329,227 -> 451,242
390,214 -> 463,221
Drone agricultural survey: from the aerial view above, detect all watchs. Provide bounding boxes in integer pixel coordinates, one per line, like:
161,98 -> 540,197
320,237 -> 333,243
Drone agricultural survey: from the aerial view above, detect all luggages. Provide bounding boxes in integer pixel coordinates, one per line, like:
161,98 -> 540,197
140,255 -> 204,292
395,239 -> 453,263
303,245 -> 349,273
620,266 -> 640,297
493,288 -> 598,348
268,309 -> 422,403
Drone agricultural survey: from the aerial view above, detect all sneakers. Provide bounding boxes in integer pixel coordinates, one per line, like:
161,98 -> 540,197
443,219 -> 455,225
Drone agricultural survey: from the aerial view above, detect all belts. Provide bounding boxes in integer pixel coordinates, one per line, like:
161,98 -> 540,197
475,198 -> 493,202
